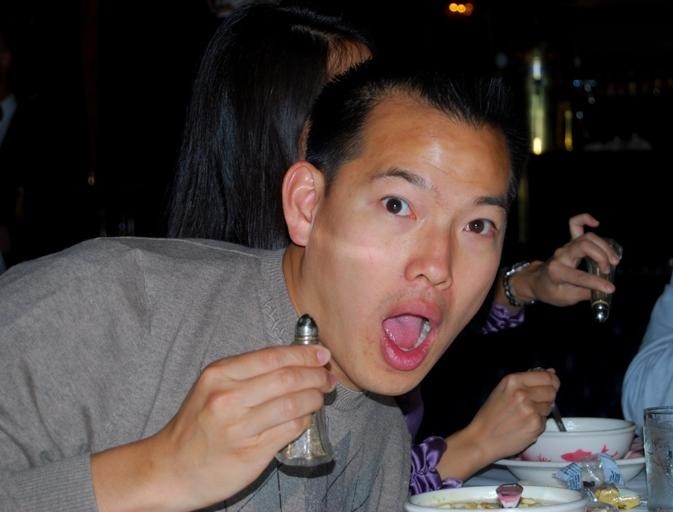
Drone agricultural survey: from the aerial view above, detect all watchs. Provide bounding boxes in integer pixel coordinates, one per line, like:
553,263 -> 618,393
502,261 -> 536,307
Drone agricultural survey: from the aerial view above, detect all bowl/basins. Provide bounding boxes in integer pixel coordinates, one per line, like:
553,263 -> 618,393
401,484 -> 589,511
520,416 -> 638,462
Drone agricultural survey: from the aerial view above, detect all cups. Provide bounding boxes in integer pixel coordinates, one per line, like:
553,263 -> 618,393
643,404 -> 672,512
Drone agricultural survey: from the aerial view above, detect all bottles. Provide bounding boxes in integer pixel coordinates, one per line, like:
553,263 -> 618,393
584,239 -> 624,324
271,313 -> 336,468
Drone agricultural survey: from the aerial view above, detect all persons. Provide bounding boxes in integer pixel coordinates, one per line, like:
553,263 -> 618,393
0,56 -> 519,512
621,269 -> 673,453
165,0 -> 621,497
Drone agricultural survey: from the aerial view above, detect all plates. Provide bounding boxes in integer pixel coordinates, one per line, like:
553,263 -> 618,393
492,449 -> 647,487
